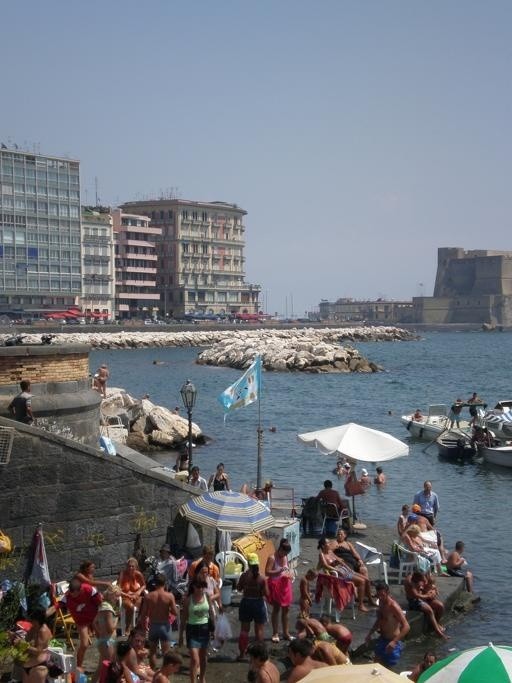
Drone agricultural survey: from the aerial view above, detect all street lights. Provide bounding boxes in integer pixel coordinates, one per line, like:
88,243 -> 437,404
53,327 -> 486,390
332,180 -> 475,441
180,378 -> 198,483
163,282 -> 169,322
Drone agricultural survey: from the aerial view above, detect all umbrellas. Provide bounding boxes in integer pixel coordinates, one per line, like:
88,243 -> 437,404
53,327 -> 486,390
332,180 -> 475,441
295,422 -> 409,525
418,640 -> 511,682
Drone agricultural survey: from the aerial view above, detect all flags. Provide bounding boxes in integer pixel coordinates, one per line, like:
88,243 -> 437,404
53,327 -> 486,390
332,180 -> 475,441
217,354 -> 261,418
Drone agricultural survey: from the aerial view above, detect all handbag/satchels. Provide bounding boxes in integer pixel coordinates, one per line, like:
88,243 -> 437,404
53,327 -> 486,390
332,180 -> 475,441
206,592 -> 216,631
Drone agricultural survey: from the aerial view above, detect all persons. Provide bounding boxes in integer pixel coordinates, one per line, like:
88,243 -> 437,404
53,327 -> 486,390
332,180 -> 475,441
449,398 -> 464,428
411,407 -> 425,422
467,391 -> 486,428
152,359 -> 165,365
94,367 -> 103,393
1,457 -> 477,681
474,426 -> 493,446
7,378 -> 34,425
173,405 -> 181,414
97,363 -> 110,396
470,424 -> 484,448
388,410 -> 395,415
176,446 -> 190,472
270,426 -> 277,432
144,392 -> 151,399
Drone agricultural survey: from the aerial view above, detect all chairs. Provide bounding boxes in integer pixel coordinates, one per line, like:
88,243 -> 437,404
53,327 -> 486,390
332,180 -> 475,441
300,487 -> 439,623
99,401 -> 128,442
49,548 -> 252,652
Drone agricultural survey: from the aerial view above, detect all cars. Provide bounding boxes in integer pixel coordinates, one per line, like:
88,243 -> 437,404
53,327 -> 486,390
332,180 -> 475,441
38,316 -> 106,325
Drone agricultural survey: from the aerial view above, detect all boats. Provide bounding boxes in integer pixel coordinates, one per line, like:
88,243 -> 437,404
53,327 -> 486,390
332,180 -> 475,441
399,395 -> 512,470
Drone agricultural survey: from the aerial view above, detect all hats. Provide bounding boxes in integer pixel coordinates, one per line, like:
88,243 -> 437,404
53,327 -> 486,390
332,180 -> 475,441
408,514 -> 417,521
159,543 -> 171,552
247,553 -> 259,566
412,504 -> 421,513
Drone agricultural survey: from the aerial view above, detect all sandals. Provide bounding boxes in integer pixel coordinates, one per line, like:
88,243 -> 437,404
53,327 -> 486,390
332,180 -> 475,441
287,636 -> 294,640
272,633 -> 279,641
